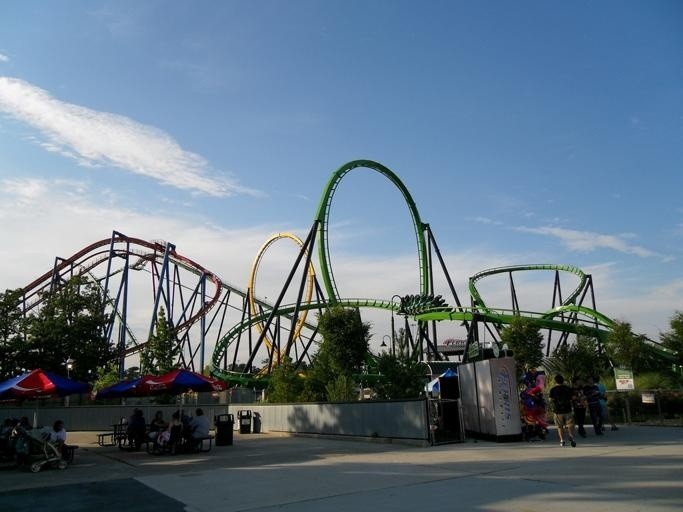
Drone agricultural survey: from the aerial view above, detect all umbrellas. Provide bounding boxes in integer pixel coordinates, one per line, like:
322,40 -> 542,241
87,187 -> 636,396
0,367 -> 93,430
134,368 -> 229,454
91,375 -> 167,397
423,367 -> 458,398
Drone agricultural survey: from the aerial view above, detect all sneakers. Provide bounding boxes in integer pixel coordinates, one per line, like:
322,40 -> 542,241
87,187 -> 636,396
559,441 -> 565,447
568,436 -> 576,448
578,424 -> 620,438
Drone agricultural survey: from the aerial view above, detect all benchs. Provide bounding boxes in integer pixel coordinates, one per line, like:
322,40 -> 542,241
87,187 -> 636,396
0,417 -> 216,462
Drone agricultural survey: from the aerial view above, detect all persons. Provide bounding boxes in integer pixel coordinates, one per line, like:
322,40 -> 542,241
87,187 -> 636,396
127,407 -> 209,456
570,376 -> 589,438
520,368 -> 546,444
582,376 -> 605,435
548,374 -> 576,447
593,375 -> 619,432
0,416 -> 67,469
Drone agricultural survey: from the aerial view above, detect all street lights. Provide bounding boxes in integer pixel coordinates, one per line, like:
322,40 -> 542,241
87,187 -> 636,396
62,355 -> 77,405
390,292 -> 406,358
380,334 -> 392,355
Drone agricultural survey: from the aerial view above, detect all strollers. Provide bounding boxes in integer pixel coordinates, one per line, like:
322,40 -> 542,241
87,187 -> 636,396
12,424 -> 69,474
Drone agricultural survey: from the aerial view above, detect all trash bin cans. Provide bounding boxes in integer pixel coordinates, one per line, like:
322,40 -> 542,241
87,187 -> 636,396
214,413 -> 234,447
237,410 -> 251,434
